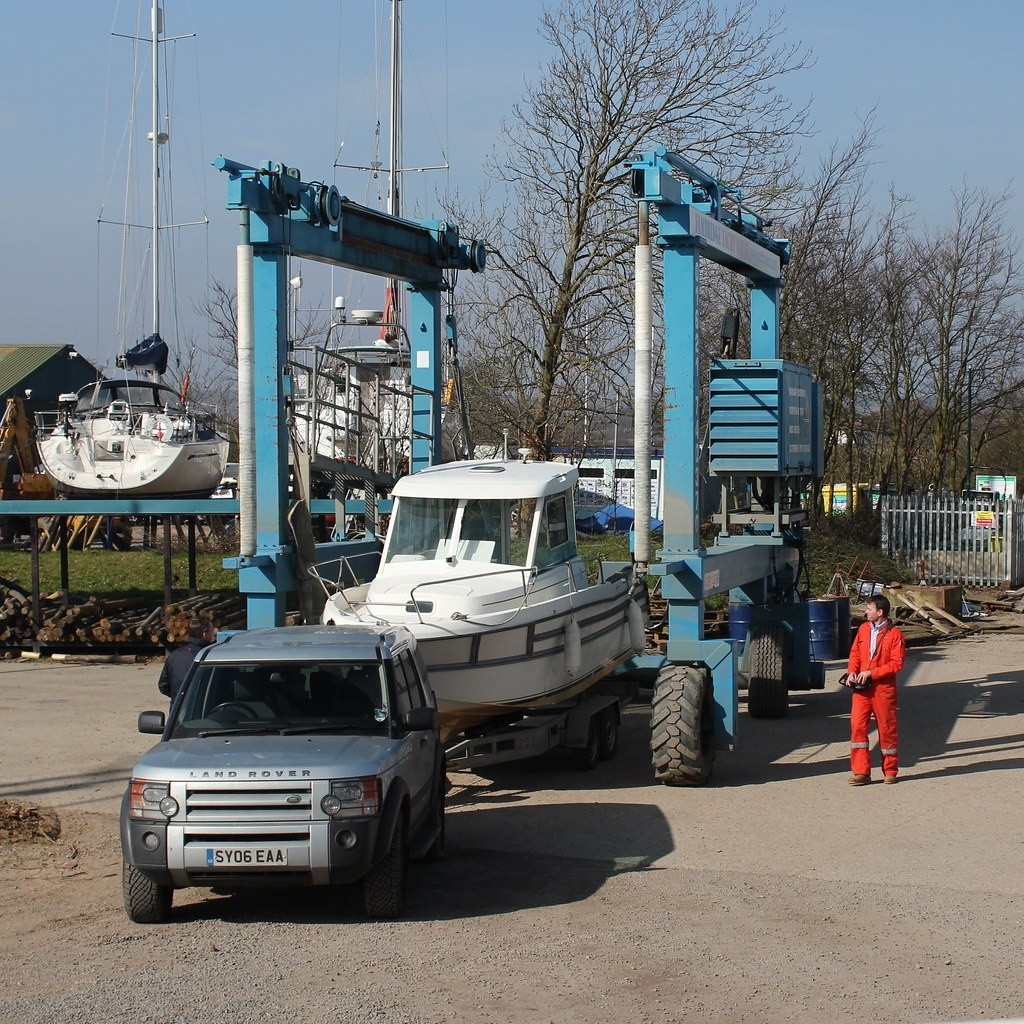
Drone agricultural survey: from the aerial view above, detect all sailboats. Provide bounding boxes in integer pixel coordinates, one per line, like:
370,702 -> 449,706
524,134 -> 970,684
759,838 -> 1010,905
34,1 -> 231,501
280,1 -> 426,501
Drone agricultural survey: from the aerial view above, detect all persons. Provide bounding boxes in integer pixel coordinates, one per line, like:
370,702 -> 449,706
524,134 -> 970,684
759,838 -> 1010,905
845,594 -> 906,786
157,616 -> 216,716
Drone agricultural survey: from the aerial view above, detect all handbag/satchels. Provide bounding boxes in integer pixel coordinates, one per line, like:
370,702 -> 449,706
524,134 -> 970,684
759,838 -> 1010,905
839,673 -> 873,690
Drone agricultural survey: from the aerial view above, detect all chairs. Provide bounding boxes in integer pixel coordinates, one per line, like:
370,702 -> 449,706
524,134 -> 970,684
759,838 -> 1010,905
214,670 -> 383,726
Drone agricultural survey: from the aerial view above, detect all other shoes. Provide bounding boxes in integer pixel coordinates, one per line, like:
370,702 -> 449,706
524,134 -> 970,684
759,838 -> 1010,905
849,774 -> 871,784
884,775 -> 896,784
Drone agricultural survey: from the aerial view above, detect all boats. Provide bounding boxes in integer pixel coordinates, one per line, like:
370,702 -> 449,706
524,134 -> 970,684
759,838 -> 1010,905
318,452 -> 647,714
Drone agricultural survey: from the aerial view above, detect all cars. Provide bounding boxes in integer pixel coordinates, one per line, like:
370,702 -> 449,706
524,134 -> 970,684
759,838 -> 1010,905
121,627 -> 451,919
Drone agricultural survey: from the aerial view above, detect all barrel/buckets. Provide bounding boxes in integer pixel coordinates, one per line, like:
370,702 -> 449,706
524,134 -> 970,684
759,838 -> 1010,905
729,602 -> 754,657
805,596 -> 852,661
